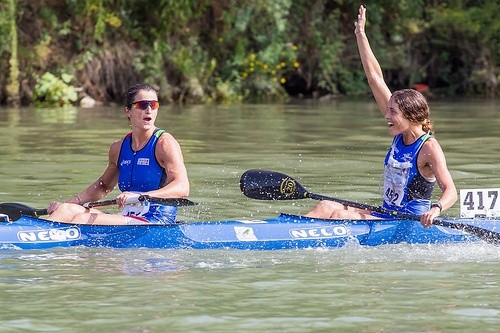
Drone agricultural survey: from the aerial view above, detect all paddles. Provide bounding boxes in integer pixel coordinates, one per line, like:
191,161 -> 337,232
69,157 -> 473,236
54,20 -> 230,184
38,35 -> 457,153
0,194 -> 200,224
239,169 -> 500,247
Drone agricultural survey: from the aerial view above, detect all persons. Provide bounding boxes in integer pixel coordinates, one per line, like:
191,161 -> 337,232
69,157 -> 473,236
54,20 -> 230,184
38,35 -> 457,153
44,83 -> 190,225
303,3 -> 458,228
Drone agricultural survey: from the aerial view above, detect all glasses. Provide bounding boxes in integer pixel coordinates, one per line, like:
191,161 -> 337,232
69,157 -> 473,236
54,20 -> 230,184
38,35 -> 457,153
127,100 -> 160,110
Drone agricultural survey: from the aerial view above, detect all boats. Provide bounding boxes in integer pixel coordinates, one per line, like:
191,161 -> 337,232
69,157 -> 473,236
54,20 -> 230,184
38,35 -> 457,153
1,191 -> 499,252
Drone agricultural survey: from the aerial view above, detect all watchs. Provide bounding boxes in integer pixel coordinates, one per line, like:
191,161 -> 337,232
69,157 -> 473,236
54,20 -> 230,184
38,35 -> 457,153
431,201 -> 442,212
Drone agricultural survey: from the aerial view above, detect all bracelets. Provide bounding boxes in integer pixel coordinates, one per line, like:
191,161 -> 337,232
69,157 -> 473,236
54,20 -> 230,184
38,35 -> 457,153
75,194 -> 83,205
355,30 -> 366,35
